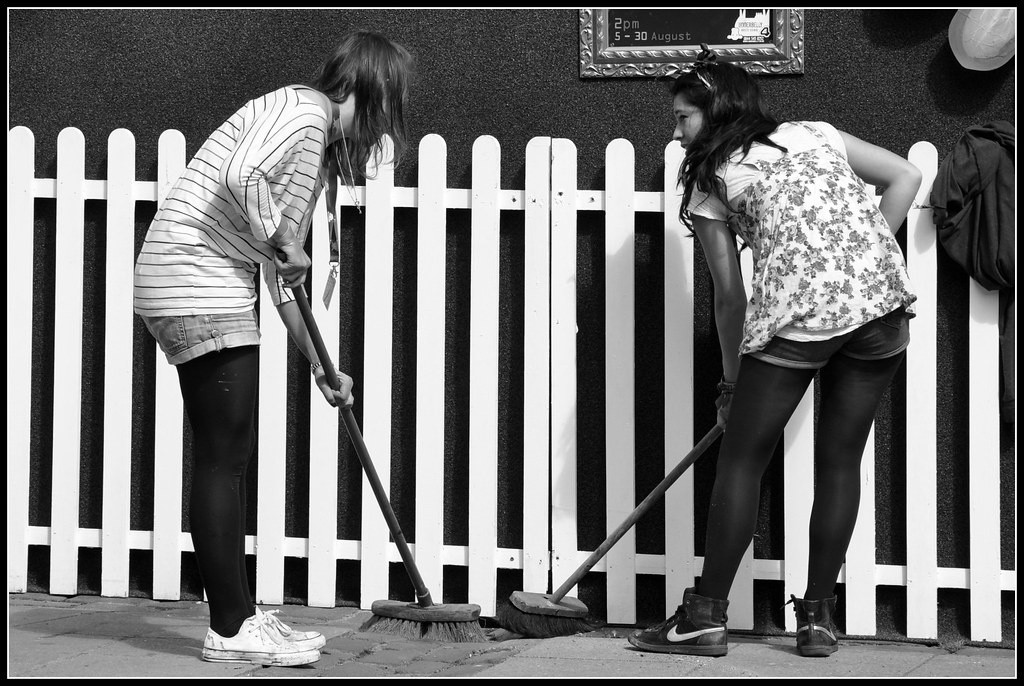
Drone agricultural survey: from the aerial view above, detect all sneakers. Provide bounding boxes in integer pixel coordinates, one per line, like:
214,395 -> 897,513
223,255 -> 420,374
782,594 -> 838,656
627,588 -> 730,656
201,605 -> 326,666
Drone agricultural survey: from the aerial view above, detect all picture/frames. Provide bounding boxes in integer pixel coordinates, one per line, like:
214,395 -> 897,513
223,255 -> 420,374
579,8 -> 806,78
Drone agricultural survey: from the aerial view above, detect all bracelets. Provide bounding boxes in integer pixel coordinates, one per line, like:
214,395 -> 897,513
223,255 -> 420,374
312,362 -> 321,374
715,376 -> 736,409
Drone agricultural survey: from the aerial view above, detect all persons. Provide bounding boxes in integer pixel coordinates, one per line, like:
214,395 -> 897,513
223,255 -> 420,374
130,31 -> 411,666
631,65 -> 924,655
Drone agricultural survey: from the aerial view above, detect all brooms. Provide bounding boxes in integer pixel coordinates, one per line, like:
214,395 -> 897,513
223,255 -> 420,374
288,279 -> 488,644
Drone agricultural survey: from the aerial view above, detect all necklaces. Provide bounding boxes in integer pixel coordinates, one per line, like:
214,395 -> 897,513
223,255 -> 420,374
332,105 -> 361,213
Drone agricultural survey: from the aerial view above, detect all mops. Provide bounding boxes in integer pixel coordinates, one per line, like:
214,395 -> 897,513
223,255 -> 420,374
496,422 -> 726,638
